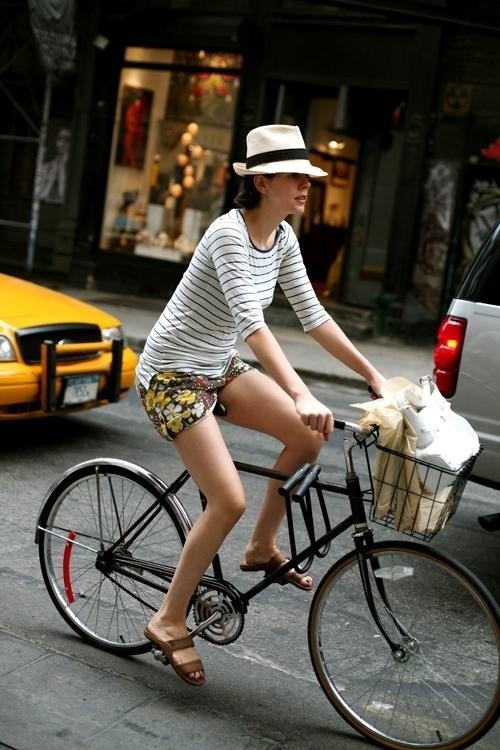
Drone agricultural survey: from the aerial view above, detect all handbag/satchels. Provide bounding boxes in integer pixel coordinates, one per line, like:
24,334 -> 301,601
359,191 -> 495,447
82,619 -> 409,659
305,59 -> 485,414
397,374 -> 483,494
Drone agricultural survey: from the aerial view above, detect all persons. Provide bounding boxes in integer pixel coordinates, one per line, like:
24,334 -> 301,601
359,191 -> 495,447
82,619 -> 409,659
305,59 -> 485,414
134,125 -> 388,685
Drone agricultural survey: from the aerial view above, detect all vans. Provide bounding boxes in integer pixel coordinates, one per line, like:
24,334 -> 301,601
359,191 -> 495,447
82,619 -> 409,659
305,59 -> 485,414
430,222 -> 500,492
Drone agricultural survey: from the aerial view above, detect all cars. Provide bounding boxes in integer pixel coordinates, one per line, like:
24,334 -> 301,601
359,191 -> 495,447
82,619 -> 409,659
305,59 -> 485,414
1,272 -> 140,422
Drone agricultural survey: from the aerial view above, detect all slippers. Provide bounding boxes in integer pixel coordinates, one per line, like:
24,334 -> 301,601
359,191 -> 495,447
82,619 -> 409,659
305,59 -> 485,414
237,551 -> 314,591
142,627 -> 207,688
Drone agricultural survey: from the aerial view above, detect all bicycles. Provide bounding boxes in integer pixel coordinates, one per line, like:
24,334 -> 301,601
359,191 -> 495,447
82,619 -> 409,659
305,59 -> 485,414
30,399 -> 500,750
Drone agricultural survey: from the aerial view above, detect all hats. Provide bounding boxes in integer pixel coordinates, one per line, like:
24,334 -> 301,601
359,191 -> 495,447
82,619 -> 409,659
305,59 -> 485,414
232,123 -> 329,177
